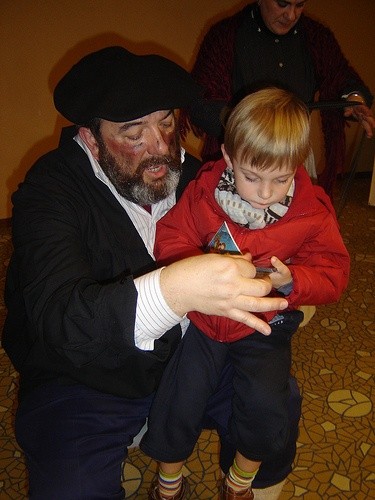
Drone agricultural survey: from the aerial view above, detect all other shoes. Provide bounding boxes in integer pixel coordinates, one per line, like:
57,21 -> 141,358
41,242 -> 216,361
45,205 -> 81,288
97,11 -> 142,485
151,476 -> 189,500
220,484 -> 254,500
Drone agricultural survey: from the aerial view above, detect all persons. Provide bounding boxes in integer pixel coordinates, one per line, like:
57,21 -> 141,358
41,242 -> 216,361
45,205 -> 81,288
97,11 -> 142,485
139,88 -> 351,500
0,47 -> 303,500
191,0 -> 375,163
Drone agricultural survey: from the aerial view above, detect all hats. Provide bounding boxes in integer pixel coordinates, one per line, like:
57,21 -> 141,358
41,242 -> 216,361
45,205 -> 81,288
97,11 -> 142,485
53,46 -> 198,122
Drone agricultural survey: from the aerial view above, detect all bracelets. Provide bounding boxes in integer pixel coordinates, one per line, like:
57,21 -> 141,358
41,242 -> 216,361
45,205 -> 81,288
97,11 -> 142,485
351,93 -> 366,105
